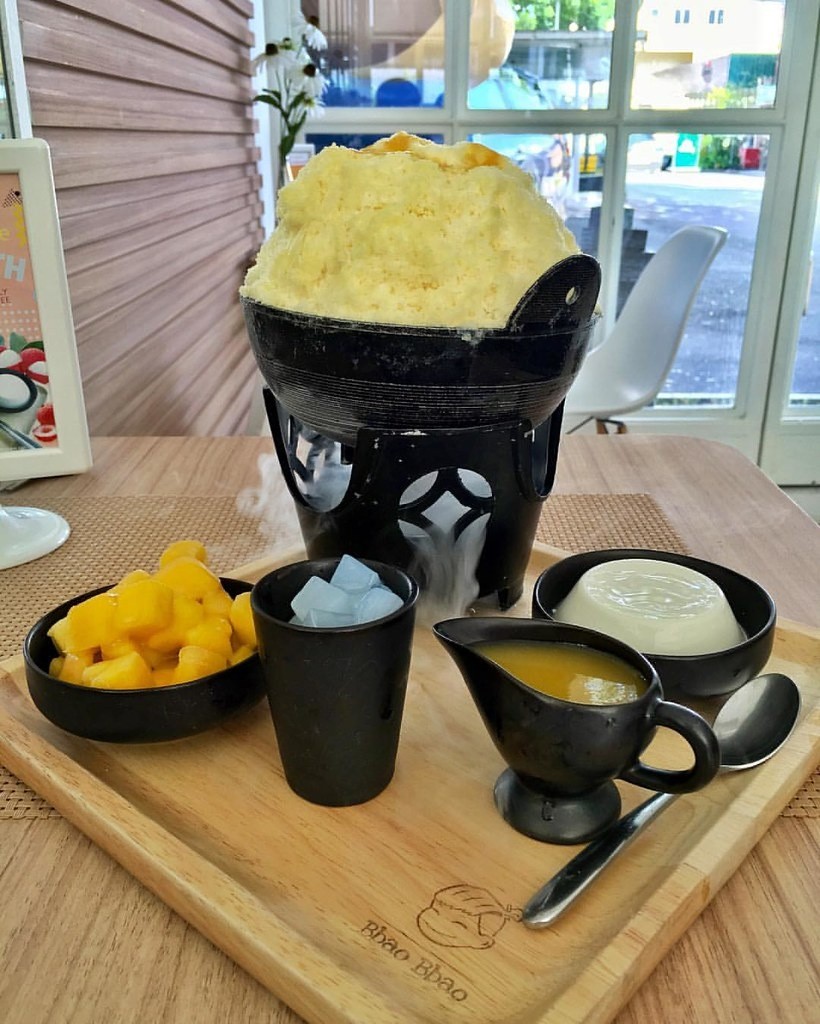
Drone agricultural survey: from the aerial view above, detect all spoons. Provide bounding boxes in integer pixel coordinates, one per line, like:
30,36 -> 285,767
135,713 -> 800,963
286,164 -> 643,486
524,673 -> 801,926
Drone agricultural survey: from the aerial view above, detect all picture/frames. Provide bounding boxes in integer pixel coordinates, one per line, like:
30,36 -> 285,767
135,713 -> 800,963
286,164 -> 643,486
0,136 -> 99,480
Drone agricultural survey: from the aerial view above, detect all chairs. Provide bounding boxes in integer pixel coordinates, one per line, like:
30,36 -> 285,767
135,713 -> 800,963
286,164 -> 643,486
560,223 -> 729,433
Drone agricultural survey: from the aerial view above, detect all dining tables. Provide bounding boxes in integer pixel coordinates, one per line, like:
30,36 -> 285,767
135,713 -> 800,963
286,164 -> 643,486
0,432 -> 820,1024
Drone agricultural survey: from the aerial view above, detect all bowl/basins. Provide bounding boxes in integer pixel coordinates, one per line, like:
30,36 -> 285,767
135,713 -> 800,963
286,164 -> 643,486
24,576 -> 270,746
241,253 -> 603,448
530,550 -> 776,702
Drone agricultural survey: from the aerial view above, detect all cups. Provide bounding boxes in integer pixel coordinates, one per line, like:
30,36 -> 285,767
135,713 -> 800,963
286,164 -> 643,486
249,557 -> 419,808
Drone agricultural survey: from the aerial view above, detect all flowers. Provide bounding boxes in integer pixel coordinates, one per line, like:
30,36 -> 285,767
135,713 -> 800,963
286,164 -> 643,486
252,14 -> 329,190
